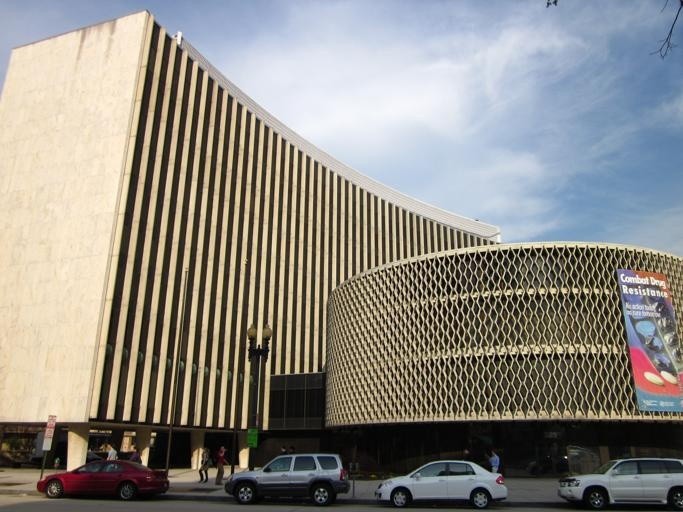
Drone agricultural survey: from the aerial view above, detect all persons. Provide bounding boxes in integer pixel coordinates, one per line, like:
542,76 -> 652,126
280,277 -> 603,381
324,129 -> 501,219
106,443 -> 117,461
199,448 -> 209,482
280,445 -> 288,455
464,447 -> 472,461
289,446 -> 296,454
130,445 -> 140,462
216,446 -> 229,484
488,448 -> 500,474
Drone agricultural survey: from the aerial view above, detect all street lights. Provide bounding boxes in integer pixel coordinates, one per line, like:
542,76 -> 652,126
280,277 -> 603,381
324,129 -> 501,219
247,323 -> 272,470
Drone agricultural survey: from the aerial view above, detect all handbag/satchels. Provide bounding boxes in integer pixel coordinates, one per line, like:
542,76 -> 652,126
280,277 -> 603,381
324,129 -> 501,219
207,459 -> 213,467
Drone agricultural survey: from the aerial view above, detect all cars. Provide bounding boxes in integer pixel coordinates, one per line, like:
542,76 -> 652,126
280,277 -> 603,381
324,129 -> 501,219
224,453 -> 350,506
557,457 -> 683,510
37,460 -> 170,500
374,459 -> 508,509
